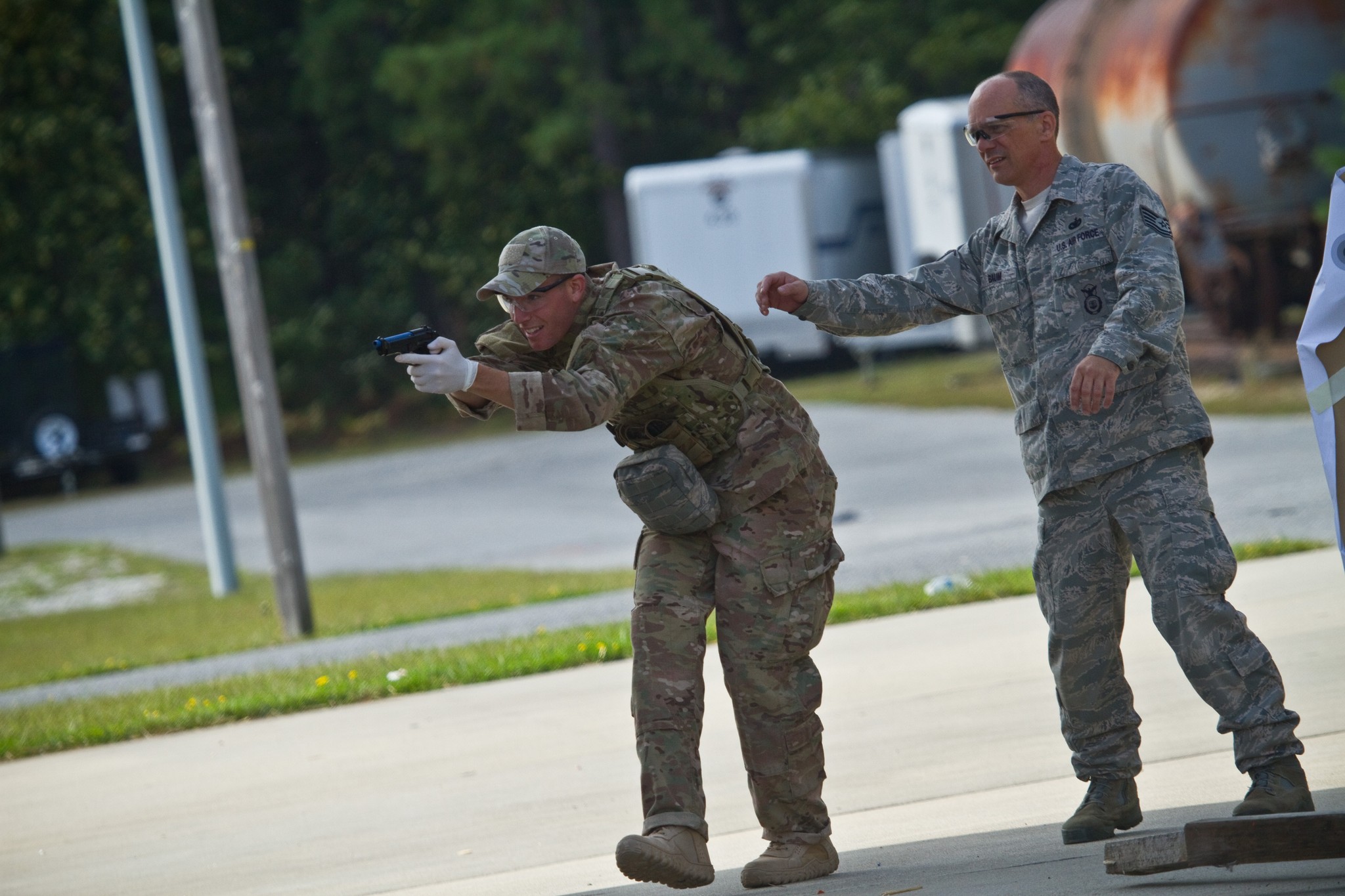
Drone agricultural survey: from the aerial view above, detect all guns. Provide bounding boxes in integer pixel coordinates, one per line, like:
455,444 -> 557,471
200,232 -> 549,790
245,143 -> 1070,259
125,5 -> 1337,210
373,325 -> 438,367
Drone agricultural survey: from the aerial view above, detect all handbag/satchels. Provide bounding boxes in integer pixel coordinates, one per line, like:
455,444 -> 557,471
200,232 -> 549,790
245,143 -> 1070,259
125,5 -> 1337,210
612,443 -> 718,535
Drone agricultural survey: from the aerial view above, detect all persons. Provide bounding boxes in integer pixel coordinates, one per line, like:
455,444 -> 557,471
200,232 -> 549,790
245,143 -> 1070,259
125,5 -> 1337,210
394,227 -> 843,890
757,73 -> 1314,848
34,411 -> 147,495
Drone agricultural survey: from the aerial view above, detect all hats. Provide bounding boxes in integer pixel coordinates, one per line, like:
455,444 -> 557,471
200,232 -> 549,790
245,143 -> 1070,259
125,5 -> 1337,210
476,225 -> 586,301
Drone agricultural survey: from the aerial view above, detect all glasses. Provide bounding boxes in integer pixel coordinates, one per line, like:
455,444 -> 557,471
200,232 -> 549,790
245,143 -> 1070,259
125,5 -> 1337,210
963,109 -> 1046,147
494,273 -> 575,314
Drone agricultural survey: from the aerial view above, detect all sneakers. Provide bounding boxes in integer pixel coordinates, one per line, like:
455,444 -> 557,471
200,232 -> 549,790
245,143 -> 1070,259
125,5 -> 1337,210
1232,754 -> 1315,816
615,825 -> 715,889
740,835 -> 839,888
1061,776 -> 1143,844
